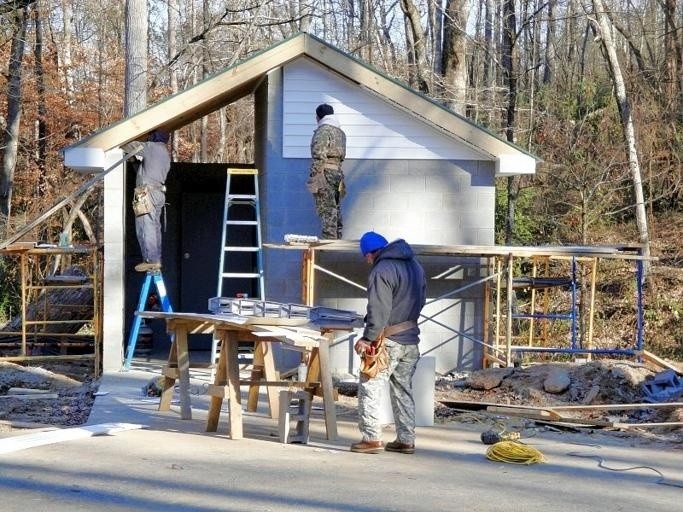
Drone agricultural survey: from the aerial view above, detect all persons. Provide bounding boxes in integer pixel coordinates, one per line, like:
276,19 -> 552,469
310,104 -> 347,241
349,231 -> 426,453
120,129 -> 171,271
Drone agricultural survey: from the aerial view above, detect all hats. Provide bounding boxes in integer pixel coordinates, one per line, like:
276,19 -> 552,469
359,231 -> 388,256
151,128 -> 170,143
315,104 -> 334,119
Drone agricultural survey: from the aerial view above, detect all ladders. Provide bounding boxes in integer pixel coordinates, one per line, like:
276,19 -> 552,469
121,268 -> 176,372
210,168 -> 265,383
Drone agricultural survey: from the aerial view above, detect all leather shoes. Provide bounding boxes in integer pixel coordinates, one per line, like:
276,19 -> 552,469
351,440 -> 385,454
385,441 -> 414,454
134,261 -> 163,272
